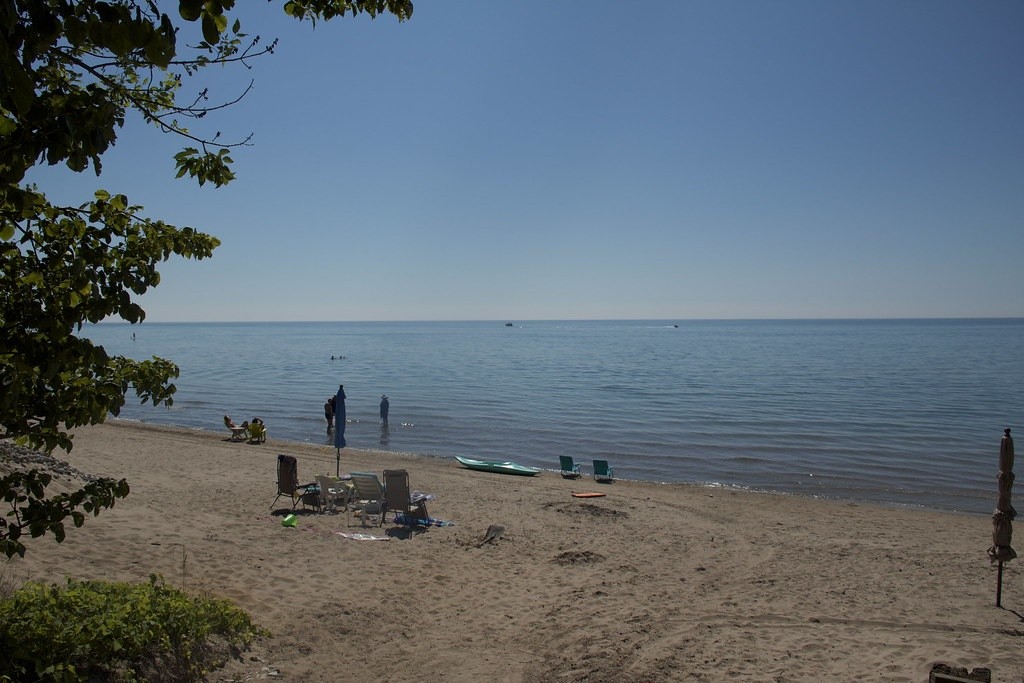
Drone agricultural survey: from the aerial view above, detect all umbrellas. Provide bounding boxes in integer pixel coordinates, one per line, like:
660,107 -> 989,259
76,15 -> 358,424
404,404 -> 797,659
986,427 -> 1017,605
333,384 -> 347,477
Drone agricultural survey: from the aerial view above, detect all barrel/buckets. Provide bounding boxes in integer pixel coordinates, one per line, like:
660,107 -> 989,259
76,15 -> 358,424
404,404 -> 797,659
282,513 -> 297,526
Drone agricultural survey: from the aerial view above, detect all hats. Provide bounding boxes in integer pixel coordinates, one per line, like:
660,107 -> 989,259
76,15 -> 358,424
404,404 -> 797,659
381,395 -> 388,398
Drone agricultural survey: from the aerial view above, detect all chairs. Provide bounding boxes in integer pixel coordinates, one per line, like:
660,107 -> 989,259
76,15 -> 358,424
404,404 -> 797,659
378,470 -> 429,529
558,456 -> 580,478
313,474 -> 353,513
348,471 -> 397,524
224,421 -> 248,440
249,423 -> 266,443
593,460 -> 613,481
268,456 -> 322,513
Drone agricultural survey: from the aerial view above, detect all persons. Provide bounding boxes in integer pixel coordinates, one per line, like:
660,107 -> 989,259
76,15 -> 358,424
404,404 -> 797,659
324,395 -> 337,435
379,395 -> 389,427
224,415 -> 265,440
